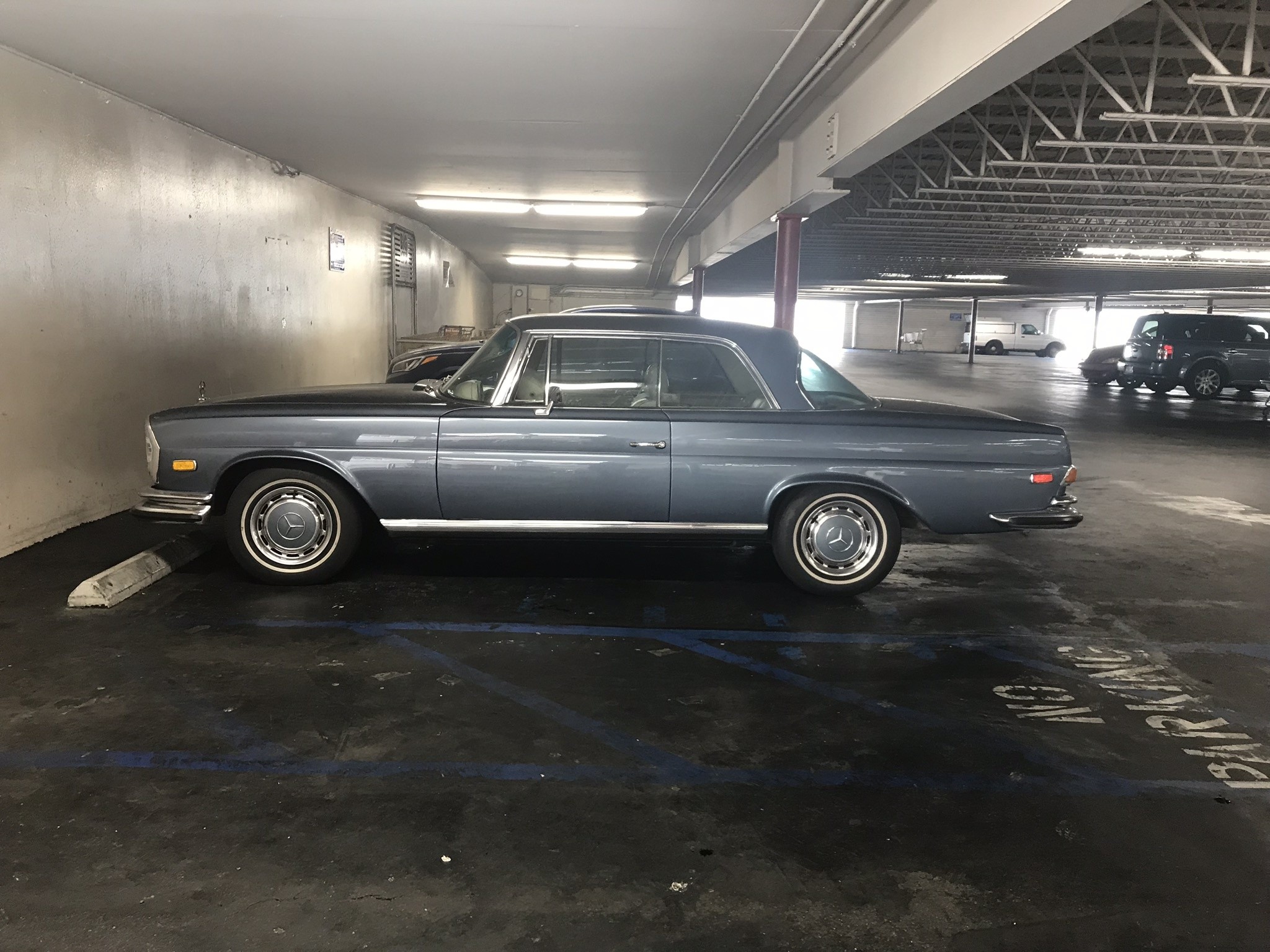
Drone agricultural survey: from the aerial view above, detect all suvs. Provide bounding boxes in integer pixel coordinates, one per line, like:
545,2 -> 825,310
1078,326 -> 1159,390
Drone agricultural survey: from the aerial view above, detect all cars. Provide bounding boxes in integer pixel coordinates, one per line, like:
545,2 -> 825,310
134,311 -> 1086,601
381,303 -> 729,385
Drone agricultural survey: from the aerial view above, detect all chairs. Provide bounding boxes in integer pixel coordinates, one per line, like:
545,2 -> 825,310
613,360 -> 673,408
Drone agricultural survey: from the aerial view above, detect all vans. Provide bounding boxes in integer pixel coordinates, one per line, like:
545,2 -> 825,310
963,320 -> 1068,358
1117,313 -> 1270,402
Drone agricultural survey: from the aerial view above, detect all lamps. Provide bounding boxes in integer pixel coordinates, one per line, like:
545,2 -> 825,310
572,258 -> 638,270
533,199 -> 649,218
413,195 -> 534,214
505,255 -> 572,266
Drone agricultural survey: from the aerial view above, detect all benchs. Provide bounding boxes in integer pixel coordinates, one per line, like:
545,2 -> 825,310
733,388 -> 775,409
